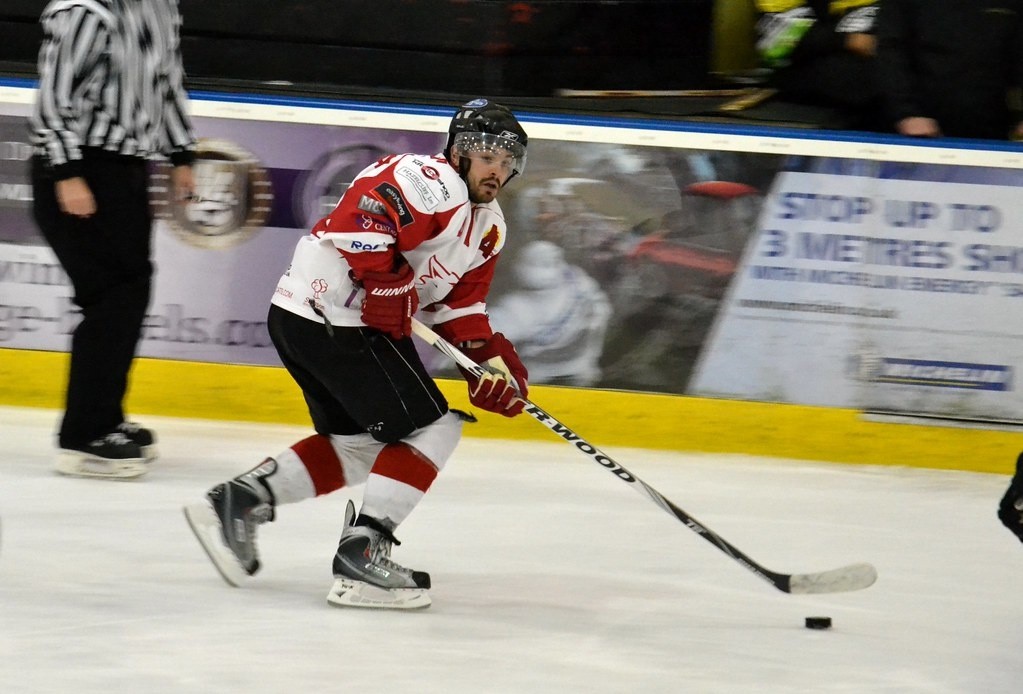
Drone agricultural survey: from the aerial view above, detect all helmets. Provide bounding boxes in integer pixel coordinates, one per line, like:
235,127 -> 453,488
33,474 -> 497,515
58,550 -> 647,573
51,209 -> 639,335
443,98 -> 528,158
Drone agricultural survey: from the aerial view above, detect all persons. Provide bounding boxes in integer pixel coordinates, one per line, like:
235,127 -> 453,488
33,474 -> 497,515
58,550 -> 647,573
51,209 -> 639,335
484,0 -> 954,140
26,1 -> 197,479
481,241 -> 613,386
183,99 -> 532,605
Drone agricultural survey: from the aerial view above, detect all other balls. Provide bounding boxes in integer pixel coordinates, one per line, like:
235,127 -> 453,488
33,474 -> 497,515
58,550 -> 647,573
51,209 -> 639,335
805,617 -> 832,630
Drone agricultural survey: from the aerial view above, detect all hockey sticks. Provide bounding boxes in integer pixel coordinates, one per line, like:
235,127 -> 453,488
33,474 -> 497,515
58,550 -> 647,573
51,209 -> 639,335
410,316 -> 876,594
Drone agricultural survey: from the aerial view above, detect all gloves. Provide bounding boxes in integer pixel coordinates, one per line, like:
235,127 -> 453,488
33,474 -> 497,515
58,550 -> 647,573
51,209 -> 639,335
360,255 -> 420,340
456,332 -> 528,418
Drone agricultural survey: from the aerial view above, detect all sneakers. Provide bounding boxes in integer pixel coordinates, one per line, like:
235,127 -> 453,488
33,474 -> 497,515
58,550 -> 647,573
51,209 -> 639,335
184,456 -> 278,588
51,433 -> 147,477
114,421 -> 160,460
327,499 -> 431,611
